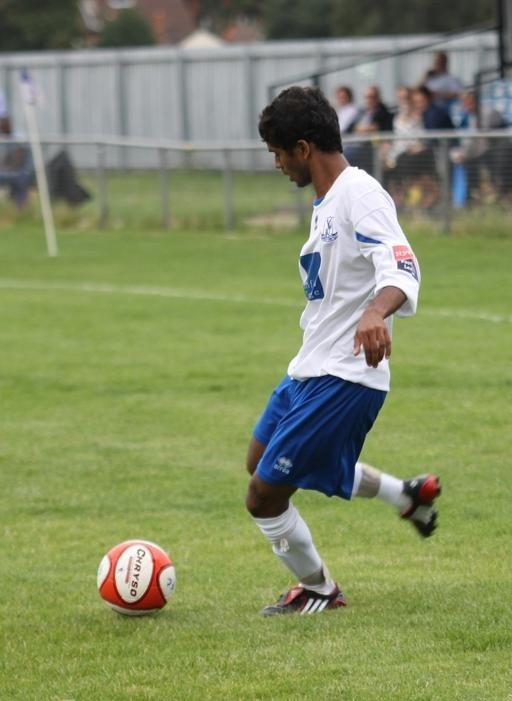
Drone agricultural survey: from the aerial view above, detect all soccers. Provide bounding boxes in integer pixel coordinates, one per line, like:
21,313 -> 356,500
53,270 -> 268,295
97,540 -> 176,617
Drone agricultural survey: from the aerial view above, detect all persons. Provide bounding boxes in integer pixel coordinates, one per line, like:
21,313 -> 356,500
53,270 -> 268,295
336,51 -> 512,211
1,112 -> 91,231
244,86 -> 441,618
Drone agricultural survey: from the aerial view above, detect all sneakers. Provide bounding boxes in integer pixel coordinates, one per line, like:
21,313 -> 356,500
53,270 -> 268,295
400,474 -> 441,537
262,583 -> 346,616
